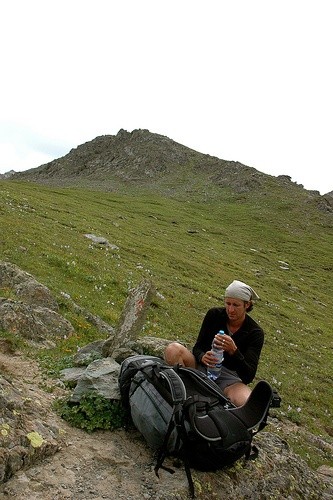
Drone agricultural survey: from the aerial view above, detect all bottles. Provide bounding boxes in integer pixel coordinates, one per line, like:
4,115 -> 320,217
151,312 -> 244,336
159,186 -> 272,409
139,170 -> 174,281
207,330 -> 224,380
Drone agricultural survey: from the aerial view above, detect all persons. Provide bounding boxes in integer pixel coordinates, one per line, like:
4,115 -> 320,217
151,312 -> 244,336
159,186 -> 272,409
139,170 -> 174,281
165,280 -> 264,407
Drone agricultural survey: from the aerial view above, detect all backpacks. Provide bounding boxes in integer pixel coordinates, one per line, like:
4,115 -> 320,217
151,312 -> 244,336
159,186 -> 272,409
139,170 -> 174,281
118,355 -> 273,471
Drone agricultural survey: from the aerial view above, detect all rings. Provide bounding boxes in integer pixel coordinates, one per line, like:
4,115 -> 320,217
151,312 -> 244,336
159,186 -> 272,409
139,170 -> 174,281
222,339 -> 225,346
